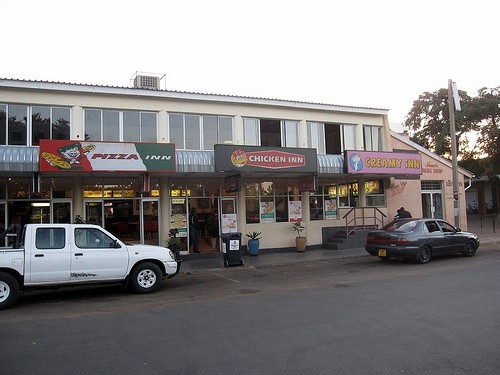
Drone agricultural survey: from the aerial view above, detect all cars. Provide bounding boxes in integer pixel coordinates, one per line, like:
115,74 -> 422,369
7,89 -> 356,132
364,217 -> 480,265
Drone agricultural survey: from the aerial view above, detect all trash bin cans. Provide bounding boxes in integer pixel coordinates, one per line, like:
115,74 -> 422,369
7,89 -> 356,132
221,232 -> 245,268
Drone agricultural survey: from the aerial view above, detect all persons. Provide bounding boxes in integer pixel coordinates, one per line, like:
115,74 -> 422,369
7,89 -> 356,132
207,206 -> 219,249
393,207 -> 412,219
186,207 -> 201,253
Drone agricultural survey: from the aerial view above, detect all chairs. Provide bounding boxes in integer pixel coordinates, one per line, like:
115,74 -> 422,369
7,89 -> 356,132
118,220 -> 159,242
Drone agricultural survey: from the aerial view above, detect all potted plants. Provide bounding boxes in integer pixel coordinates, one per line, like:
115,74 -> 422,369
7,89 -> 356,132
168,229 -> 182,256
294,221 -> 307,252
245,231 -> 263,254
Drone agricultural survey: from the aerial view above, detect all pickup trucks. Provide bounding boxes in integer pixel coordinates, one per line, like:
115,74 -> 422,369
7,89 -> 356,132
0,223 -> 184,311
